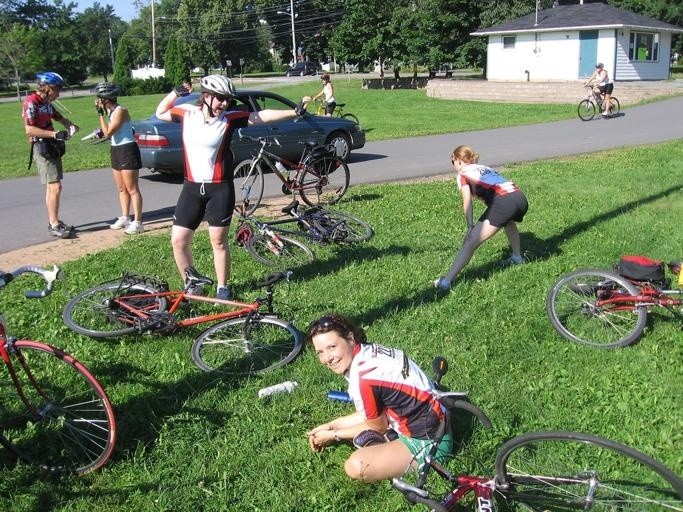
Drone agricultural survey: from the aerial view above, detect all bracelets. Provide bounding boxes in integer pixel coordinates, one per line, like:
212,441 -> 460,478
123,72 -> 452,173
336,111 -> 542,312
333,427 -> 342,441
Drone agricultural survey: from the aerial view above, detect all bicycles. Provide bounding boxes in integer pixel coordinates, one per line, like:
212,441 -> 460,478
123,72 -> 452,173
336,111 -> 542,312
577,83 -> 620,121
234,127 -> 352,218
0,264 -> 120,480
544,253 -> 683,352
312,97 -> 360,124
235,200 -> 374,265
65,266 -> 302,379
384,357 -> 683,511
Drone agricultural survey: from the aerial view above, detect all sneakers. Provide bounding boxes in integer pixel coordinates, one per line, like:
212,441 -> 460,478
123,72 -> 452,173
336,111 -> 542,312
434,273 -> 454,291
124,221 -> 145,236
216,283 -> 236,307
110,215 -> 132,230
500,253 -> 525,267
595,99 -> 603,105
183,283 -> 208,303
601,110 -> 613,116
47,220 -> 77,239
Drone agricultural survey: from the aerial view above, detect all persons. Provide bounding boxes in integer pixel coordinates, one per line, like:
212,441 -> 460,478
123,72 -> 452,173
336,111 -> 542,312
392,68 -> 399,80
303,316 -> 452,483
313,74 -> 335,118
92,82 -> 145,235
431,143 -> 529,290
21,71 -> 78,242
157,72 -> 311,306
584,63 -> 614,118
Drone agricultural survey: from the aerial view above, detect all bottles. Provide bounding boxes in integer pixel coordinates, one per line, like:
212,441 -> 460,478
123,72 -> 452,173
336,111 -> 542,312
326,391 -> 351,404
257,381 -> 298,398
274,162 -> 289,178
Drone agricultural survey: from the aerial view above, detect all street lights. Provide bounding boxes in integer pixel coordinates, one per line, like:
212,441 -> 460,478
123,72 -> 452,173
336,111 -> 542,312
148,16 -> 165,68
276,11 -> 297,65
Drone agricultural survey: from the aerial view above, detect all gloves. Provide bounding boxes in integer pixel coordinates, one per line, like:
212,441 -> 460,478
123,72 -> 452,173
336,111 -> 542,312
174,82 -> 192,98
68,124 -> 80,137
95,99 -> 109,117
54,130 -> 70,142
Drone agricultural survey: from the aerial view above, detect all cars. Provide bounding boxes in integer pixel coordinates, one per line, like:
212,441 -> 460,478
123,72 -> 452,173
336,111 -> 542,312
131,90 -> 366,178
141,54 -> 162,69
285,62 -> 317,78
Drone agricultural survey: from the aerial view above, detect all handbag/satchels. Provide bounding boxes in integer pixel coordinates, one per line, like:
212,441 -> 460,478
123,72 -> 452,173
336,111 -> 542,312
36,138 -> 67,161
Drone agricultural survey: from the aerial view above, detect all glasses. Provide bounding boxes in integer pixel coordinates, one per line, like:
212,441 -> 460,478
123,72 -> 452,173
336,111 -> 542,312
214,95 -> 233,103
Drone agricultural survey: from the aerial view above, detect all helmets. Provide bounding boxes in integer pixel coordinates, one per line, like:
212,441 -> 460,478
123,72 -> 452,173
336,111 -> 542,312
95,79 -> 120,100
595,63 -> 604,68
320,73 -> 330,80
200,73 -> 237,97
34,71 -> 69,88
